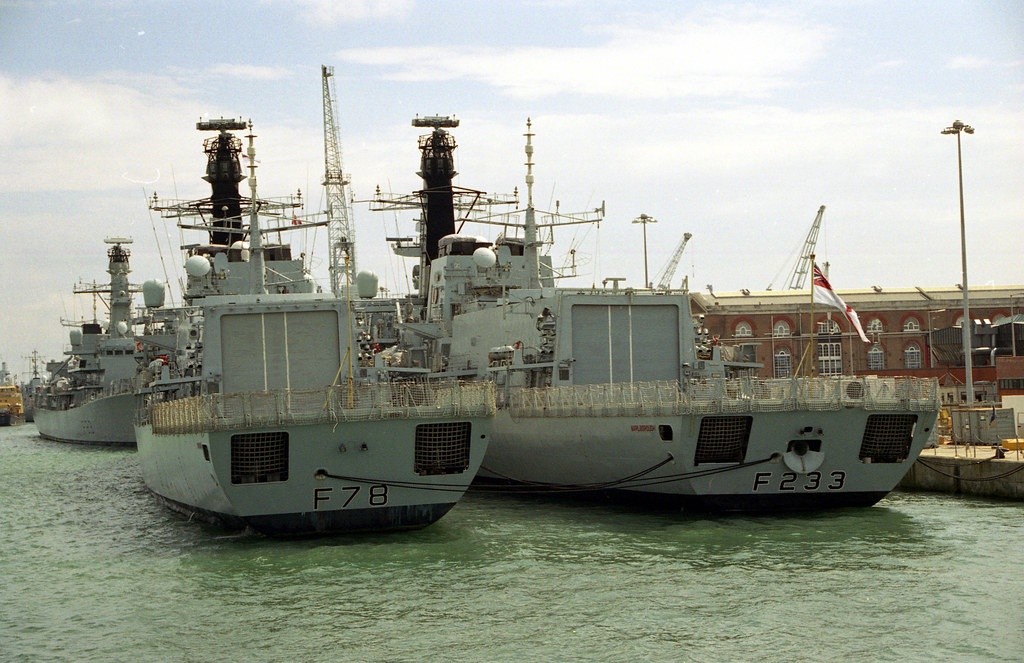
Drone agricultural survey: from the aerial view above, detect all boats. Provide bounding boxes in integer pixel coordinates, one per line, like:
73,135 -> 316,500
0,63 -> 943,542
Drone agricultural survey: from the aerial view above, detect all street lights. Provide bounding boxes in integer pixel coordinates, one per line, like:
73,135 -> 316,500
940,119 -> 977,405
